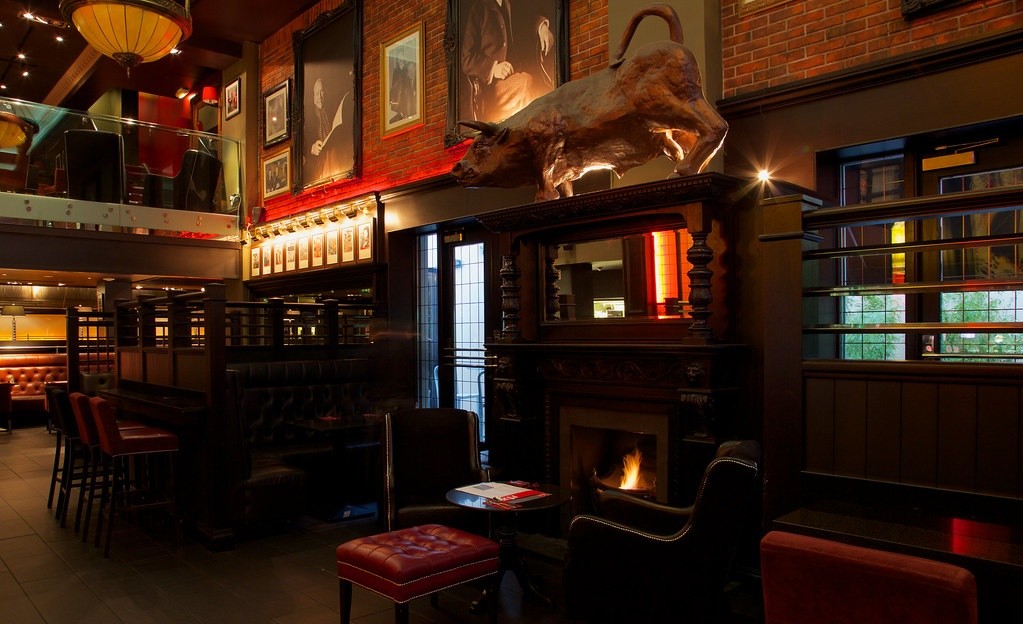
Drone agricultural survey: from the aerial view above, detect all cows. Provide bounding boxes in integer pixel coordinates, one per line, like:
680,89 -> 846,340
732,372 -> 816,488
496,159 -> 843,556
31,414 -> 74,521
449,7 -> 733,201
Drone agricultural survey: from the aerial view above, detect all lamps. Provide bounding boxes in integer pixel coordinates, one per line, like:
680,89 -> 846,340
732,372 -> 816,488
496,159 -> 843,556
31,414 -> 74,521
286,217 -> 303,233
202,83 -> 223,109
261,223 -> 274,239
58,0 -> 193,80
328,206 -> 346,223
240,231 -> 252,246
251,227 -> 262,242
300,212 -> 314,229
313,209 -> 329,226
273,221 -> 286,236
346,201 -> 369,219
174,88 -> 190,102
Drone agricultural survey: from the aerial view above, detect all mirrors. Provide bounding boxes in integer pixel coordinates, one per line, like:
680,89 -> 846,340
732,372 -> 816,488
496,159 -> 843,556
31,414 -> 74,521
537,220 -> 693,327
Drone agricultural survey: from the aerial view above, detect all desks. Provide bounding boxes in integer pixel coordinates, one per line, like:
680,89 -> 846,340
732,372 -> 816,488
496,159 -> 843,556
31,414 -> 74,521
284,415 -> 384,524
770,497 -> 1023,624
446,480 -> 573,615
96,388 -> 208,429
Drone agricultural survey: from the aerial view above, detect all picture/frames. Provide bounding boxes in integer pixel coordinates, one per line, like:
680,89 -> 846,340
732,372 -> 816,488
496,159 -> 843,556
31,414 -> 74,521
262,77 -> 291,150
379,20 -> 426,140
310,230 -> 325,270
291,0 -> 364,197
340,225 -> 357,265
297,234 -> 310,273
224,76 -> 241,121
442,0 -> 571,150
261,146 -> 291,203
250,237 -> 297,279
324,227 -> 340,269
357,220 -> 373,264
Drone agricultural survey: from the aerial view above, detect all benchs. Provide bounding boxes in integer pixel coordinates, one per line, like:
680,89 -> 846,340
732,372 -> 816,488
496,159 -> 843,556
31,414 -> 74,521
227,358 -> 368,464
0,352 -> 114,430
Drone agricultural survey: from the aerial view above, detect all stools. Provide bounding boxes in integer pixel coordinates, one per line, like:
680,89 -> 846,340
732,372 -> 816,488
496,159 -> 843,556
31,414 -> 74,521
335,524 -> 502,624
44,385 -> 185,560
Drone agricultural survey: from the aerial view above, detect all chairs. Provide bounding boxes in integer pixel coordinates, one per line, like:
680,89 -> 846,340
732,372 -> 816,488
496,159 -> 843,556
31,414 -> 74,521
224,368 -> 306,542
560,439 -> 763,624
142,148 -> 222,212
0,382 -> 13,435
759,529 -> 979,624
383,408 -> 495,540
64,129 -> 130,204
